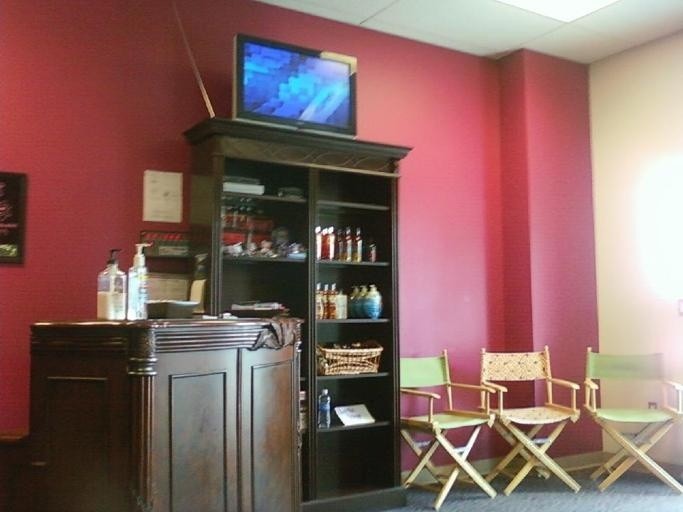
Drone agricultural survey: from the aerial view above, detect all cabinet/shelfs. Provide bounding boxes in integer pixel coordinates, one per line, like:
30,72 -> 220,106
314,281 -> 383,321
222,198 -> 273,253
316,226 -> 376,263
318,389 -> 331,429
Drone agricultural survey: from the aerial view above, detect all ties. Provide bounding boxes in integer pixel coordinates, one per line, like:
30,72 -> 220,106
231,33 -> 357,140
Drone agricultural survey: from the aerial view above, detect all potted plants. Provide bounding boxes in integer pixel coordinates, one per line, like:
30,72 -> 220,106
0,169 -> 27,265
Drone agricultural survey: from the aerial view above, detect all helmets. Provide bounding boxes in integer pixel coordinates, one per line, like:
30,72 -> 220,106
146,300 -> 200,318
229,309 -> 282,319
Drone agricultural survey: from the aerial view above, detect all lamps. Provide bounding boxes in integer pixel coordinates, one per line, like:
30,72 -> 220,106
398,348 -> 497,510
578,347 -> 682,496
481,346 -> 583,499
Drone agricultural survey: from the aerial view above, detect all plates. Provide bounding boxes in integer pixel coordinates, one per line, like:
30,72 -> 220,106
31,319 -> 301,512
182,117 -> 414,511
